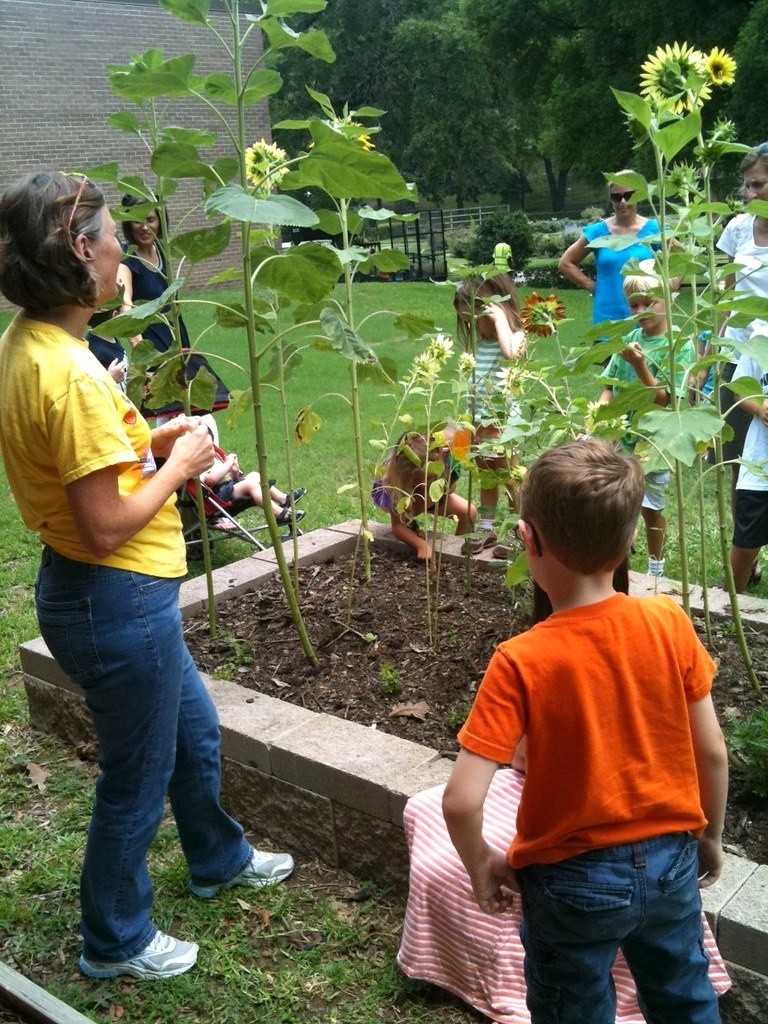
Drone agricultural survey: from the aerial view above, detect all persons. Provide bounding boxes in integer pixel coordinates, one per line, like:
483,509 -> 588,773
1,172 -> 295,981
442,438 -> 728,1024
187,424 -> 308,526
689,141 -> 768,582
396,559 -> 733,1024
558,169 -> 689,368
576,258 -> 696,578
85,286 -> 128,395
371,431 -> 477,572
453,269 -> 527,558
116,192 -> 191,426
726,322 -> 768,594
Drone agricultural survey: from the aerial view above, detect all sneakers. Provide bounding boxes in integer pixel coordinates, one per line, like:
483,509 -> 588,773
493,534 -> 524,558
79,928 -> 199,981
461,528 -> 498,553
190,846 -> 294,897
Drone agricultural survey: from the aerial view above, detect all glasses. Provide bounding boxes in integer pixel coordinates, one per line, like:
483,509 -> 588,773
58,171 -> 89,243
611,189 -> 635,203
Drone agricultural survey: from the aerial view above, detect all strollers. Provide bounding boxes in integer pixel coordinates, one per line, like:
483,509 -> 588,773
115,354 -> 305,560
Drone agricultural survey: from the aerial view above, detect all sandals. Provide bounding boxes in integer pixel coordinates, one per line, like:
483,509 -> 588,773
280,486 -> 306,508
714,559 -> 762,594
276,508 -> 306,526
